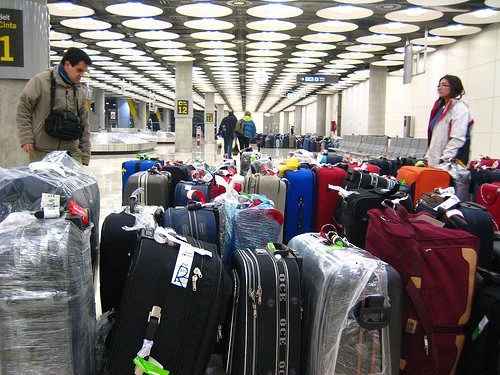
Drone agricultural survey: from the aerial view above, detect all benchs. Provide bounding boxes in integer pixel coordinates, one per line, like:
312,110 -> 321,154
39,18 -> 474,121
328,136 -> 428,161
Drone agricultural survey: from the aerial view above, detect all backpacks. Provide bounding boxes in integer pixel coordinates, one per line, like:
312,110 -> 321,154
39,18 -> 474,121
220,117 -> 233,138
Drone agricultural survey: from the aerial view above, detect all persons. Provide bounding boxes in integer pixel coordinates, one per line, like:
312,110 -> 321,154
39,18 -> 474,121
423,75 -> 474,168
219,110 -> 238,159
234,111 -> 256,160
15,47 -> 92,166
290,126 -> 294,135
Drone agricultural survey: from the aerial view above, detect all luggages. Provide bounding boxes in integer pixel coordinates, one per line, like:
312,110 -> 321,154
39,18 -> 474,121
0,209 -> 101,374
100,150 -> 500,374
0,150 -> 98,284
249,133 -> 335,153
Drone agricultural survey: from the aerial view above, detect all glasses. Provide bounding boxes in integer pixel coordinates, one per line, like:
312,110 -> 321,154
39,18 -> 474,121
437,85 -> 451,88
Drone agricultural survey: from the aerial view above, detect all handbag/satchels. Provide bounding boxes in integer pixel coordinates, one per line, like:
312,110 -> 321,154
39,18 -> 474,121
44,108 -> 84,142
233,119 -> 244,135
232,138 -> 239,156
